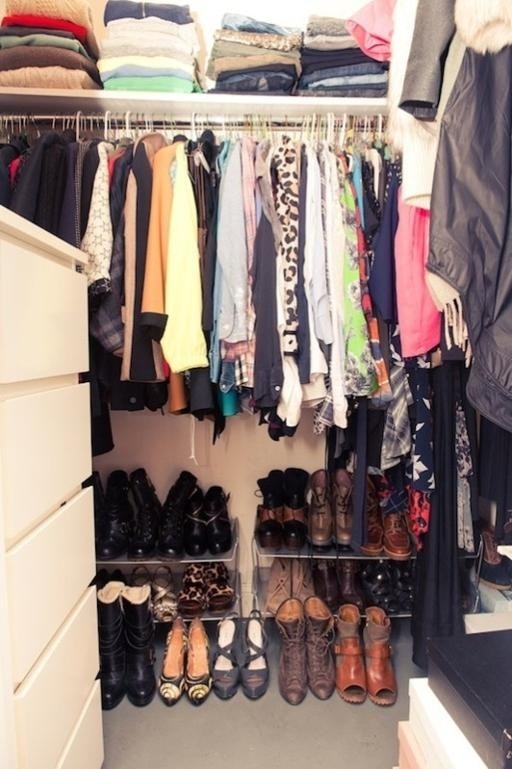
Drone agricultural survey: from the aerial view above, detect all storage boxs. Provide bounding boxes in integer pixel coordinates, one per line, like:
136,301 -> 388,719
391,627 -> 512,769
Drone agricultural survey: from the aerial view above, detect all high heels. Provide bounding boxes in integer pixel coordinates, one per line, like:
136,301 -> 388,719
157,616 -> 189,707
184,616 -> 213,707
203,486 -> 232,556
241,610 -> 269,699
180,484 -> 207,557
212,612 -> 241,700
465,518 -> 511,591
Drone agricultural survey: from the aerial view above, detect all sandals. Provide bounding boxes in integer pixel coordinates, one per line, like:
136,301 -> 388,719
202,561 -> 235,618
387,560 -> 417,611
361,561 -> 401,614
151,566 -> 178,623
176,563 -> 205,619
131,566 -> 150,586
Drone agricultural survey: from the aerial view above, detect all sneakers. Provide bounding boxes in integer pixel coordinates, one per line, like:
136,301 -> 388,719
109,568 -> 126,585
89,568 -> 109,591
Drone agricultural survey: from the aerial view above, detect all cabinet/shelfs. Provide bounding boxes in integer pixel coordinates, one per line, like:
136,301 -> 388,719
0,206 -> 105,768
251,535 -> 485,622
95,516 -> 247,652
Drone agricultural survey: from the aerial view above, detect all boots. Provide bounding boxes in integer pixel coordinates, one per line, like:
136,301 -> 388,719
362,606 -> 398,706
309,559 -> 340,612
158,471 -> 197,563
257,470 -> 285,551
303,596 -> 335,700
309,469 -> 333,553
118,582 -> 156,707
266,558 -> 290,615
361,467 -> 390,556
332,468 -> 352,552
275,597 -> 308,706
333,604 -> 367,703
378,505 -> 412,562
282,468 -> 309,552
127,468 -> 162,561
96,471 -> 128,561
336,560 -> 367,615
97,581 -> 125,709
291,559 -> 316,606
